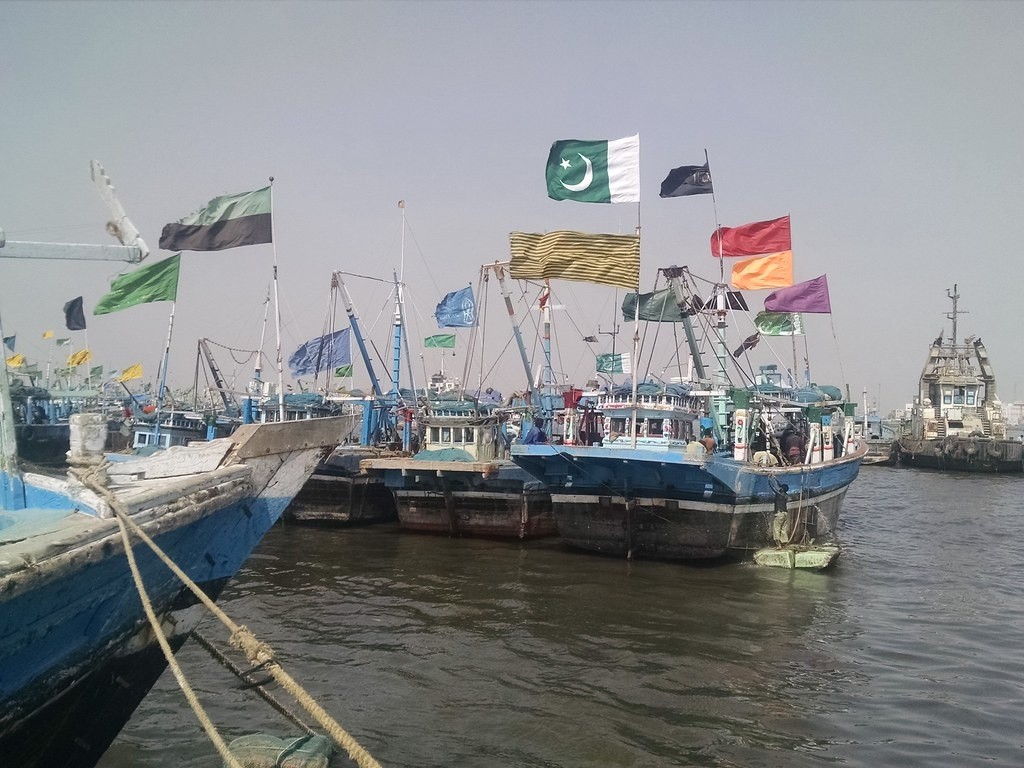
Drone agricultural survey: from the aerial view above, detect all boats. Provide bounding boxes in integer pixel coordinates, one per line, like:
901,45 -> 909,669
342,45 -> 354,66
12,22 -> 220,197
509,262 -> 869,563
357,261 -> 601,541
0,413 -> 365,768
0,264 -> 430,526
890,282 -> 1024,473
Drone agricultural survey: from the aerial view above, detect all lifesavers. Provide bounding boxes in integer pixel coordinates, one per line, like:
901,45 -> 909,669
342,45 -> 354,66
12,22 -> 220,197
21,424 -> 37,443
964,439 -> 980,455
987,440 -> 1004,458
943,436 -> 959,452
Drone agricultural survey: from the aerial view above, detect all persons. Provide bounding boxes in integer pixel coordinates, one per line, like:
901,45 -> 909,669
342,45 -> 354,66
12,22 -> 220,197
686,429 -> 716,455
782,425 -> 804,463
768,474 -> 789,549
523,419 -> 548,444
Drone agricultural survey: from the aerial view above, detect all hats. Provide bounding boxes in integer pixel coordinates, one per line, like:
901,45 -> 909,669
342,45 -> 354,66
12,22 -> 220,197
780,484 -> 789,491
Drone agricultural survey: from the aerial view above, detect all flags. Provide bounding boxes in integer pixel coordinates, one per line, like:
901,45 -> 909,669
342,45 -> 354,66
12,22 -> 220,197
336,364 -> 352,377
734,276 -> 830,358
711,215 -> 791,256
290,326 -> 350,376
510,232 -> 639,288
547,136 -> 639,205
94,254 -> 181,314
436,287 -> 480,328
4,330 -> 143,383
159,186 -> 273,250
660,161 -> 712,198
623,292 -> 747,324
424,334 -> 456,348
733,251 -> 793,290
63,297 -> 85,331
597,352 -> 631,374
